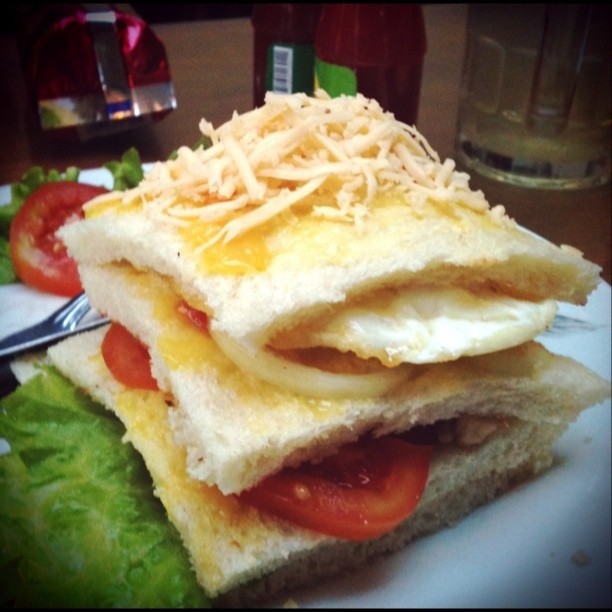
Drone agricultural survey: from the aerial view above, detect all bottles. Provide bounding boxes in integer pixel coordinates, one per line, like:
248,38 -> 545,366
313,1 -> 428,131
252,0 -> 311,114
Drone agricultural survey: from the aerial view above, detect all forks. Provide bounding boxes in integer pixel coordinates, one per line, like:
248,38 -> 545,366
0,292 -> 90,354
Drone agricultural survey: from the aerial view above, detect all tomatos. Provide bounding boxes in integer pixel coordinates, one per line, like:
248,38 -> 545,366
2,146 -> 143,300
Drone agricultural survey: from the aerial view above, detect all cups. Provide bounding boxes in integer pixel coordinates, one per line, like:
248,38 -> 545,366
456,0 -> 610,195
